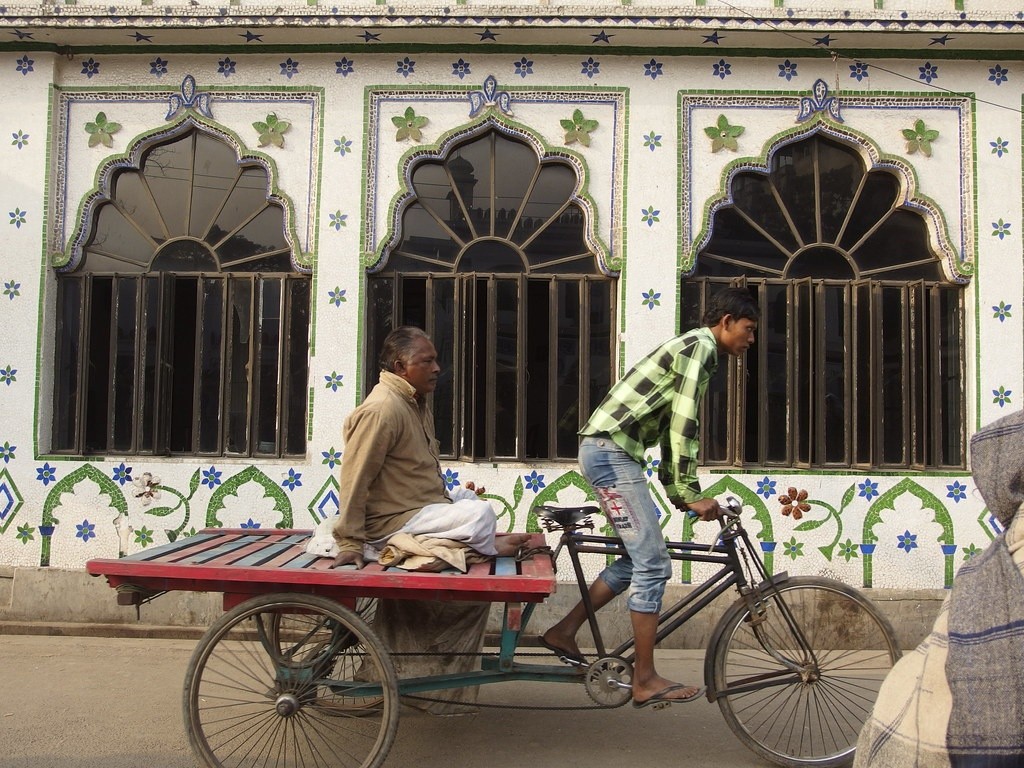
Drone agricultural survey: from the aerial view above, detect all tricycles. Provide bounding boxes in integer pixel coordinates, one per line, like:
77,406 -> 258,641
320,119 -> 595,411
86,495 -> 904,768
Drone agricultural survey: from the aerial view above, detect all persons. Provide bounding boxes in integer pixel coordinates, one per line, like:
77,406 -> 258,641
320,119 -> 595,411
331,326 -> 532,572
537,287 -> 763,709
852,409 -> 1024,768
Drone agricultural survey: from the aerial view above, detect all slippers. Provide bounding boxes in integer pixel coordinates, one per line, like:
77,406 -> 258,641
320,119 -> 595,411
538,634 -> 591,668
632,683 -> 704,710
